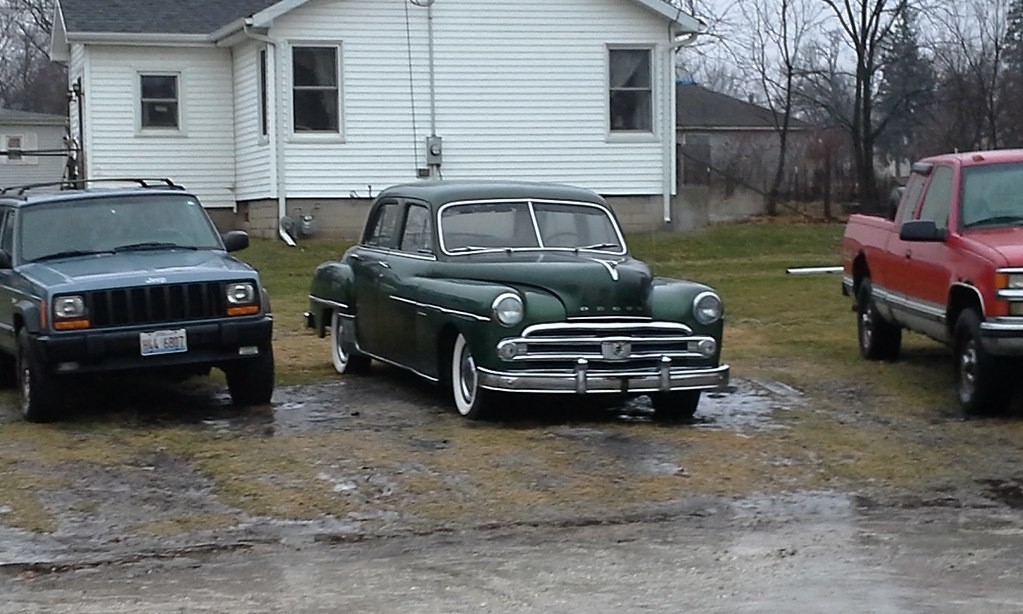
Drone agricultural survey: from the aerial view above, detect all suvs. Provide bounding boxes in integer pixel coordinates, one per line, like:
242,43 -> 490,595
0,175 -> 276,422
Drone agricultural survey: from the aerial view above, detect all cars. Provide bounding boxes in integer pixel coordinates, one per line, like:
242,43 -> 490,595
301,178 -> 731,424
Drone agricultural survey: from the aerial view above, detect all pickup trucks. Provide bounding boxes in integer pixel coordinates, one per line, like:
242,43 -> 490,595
840,149 -> 1022,416
